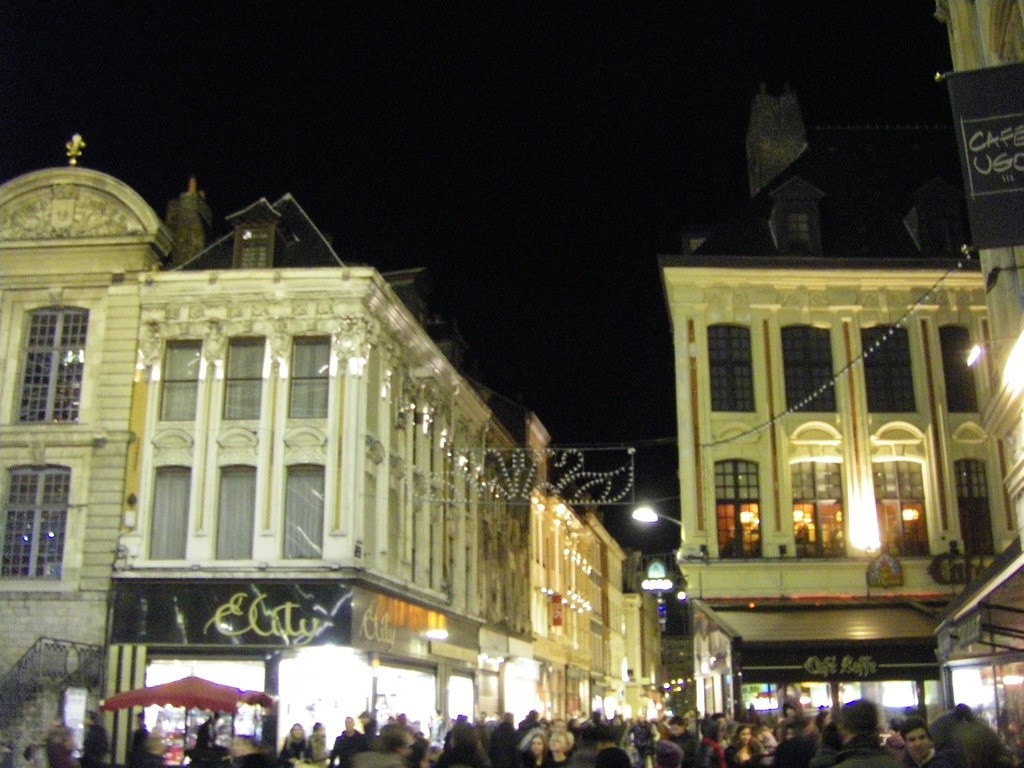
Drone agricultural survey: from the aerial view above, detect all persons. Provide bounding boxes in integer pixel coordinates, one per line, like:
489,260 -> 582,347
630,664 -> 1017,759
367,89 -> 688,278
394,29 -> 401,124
46,698 -> 1006,768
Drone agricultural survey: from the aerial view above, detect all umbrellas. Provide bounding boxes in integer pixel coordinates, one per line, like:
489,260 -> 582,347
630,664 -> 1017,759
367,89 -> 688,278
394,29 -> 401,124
98,676 -> 274,751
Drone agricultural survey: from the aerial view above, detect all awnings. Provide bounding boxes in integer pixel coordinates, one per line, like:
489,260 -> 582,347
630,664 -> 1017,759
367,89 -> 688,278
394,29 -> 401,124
933,530 -> 1024,653
